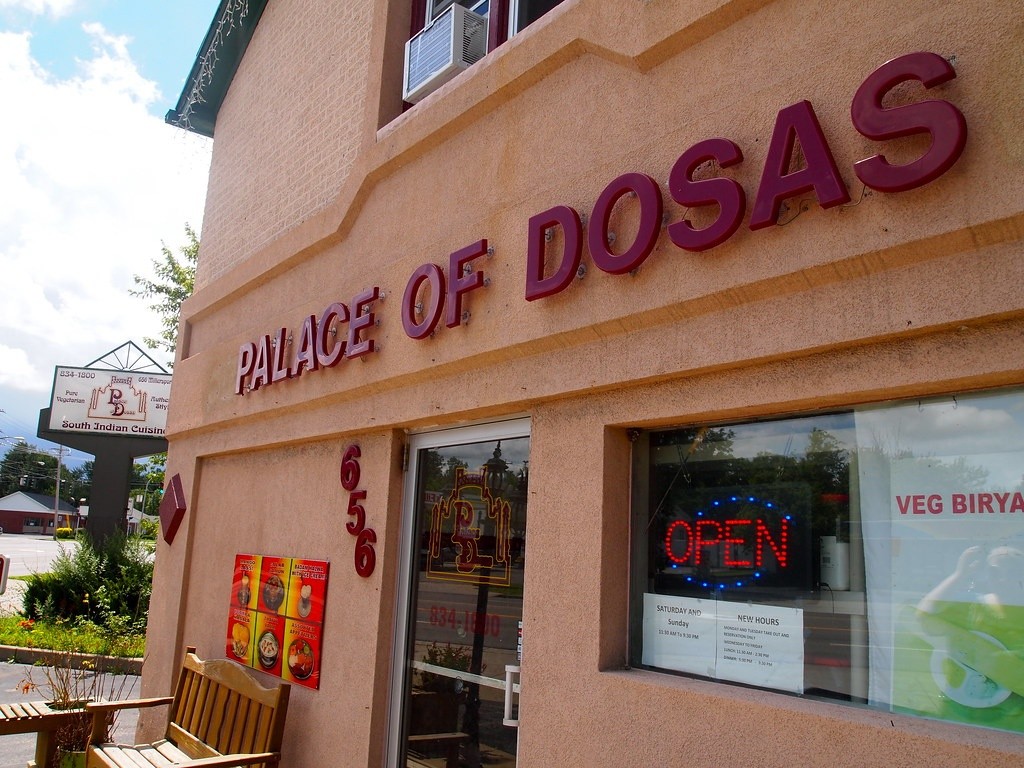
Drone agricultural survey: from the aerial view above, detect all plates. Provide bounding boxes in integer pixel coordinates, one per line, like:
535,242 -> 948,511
930,630 -> 1015,707
237,587 -> 252,605
262,576 -> 285,610
287,638 -> 314,680
230,624 -> 248,657
258,630 -> 279,669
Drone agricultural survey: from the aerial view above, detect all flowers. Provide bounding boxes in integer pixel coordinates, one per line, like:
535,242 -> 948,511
13,618 -> 149,750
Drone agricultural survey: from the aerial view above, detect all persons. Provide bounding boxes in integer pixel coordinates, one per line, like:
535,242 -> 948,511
914,546 -> 1024,733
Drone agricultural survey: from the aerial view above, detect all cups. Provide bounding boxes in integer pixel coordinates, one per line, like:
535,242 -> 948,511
300,586 -> 311,609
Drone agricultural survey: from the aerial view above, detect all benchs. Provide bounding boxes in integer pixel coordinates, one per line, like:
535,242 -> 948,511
85,646 -> 291,768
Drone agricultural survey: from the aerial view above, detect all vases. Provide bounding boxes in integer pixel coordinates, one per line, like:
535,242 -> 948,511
51,745 -> 95,767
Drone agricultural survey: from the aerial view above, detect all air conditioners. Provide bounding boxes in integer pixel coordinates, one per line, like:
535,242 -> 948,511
402,3 -> 487,106
